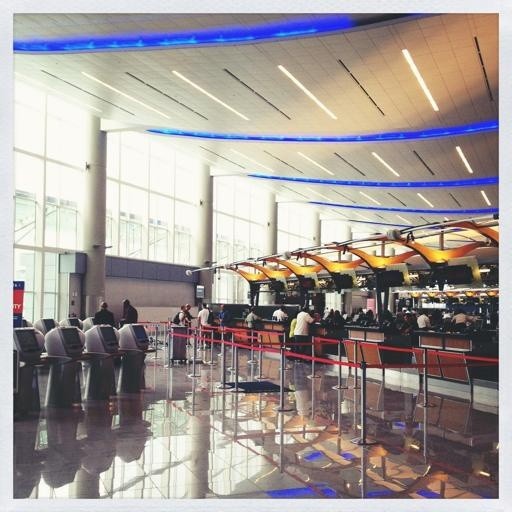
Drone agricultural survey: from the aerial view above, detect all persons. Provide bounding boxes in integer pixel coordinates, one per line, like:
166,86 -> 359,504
122,299 -> 138,324
95,302 -> 115,327
179,302 -> 473,362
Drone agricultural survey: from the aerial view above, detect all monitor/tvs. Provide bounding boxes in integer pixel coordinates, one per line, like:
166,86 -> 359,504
100,324 -> 118,346
338,273 -> 354,289
382,269 -> 404,287
300,277 -> 315,291
132,325 -> 148,341
43,318 -> 55,332
61,327 -> 82,353
93,318 -> 96,325
273,280 -> 284,290
14,328 -> 41,354
68,318 -> 81,328
437,265 -> 474,284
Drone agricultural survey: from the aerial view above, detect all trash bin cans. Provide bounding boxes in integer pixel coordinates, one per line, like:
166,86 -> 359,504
169,327 -> 187,365
